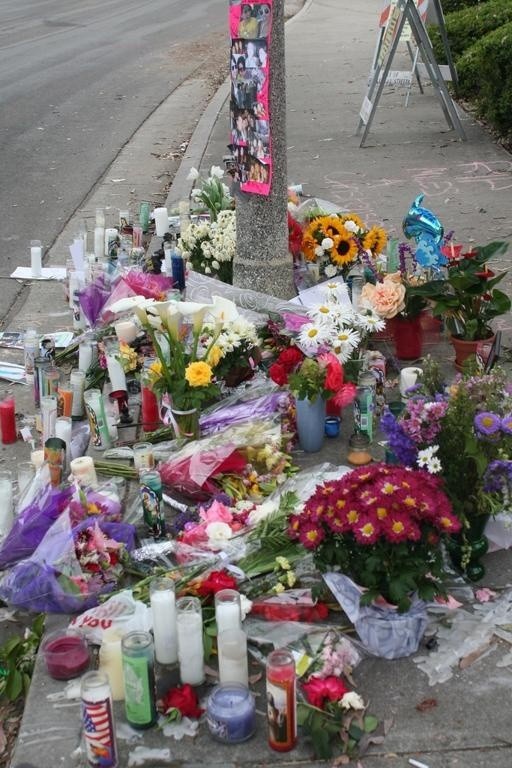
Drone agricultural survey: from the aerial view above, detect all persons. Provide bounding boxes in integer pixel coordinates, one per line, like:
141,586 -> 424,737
227,1 -> 271,182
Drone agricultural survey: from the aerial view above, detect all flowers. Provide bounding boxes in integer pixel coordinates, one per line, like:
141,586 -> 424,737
1,160 -> 511,768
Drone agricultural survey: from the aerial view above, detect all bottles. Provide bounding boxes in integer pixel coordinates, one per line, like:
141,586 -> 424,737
30,201 -> 185,300
216,629 -> 250,691
175,596 -> 204,687
213,588 -> 242,631
205,680 -> 257,743
150,577 -> 178,666
345,434 -> 372,465
100,628 -> 123,702
0,319 -> 167,540
81,669 -> 120,767
120,631 -> 158,730
265,651 -> 299,753
